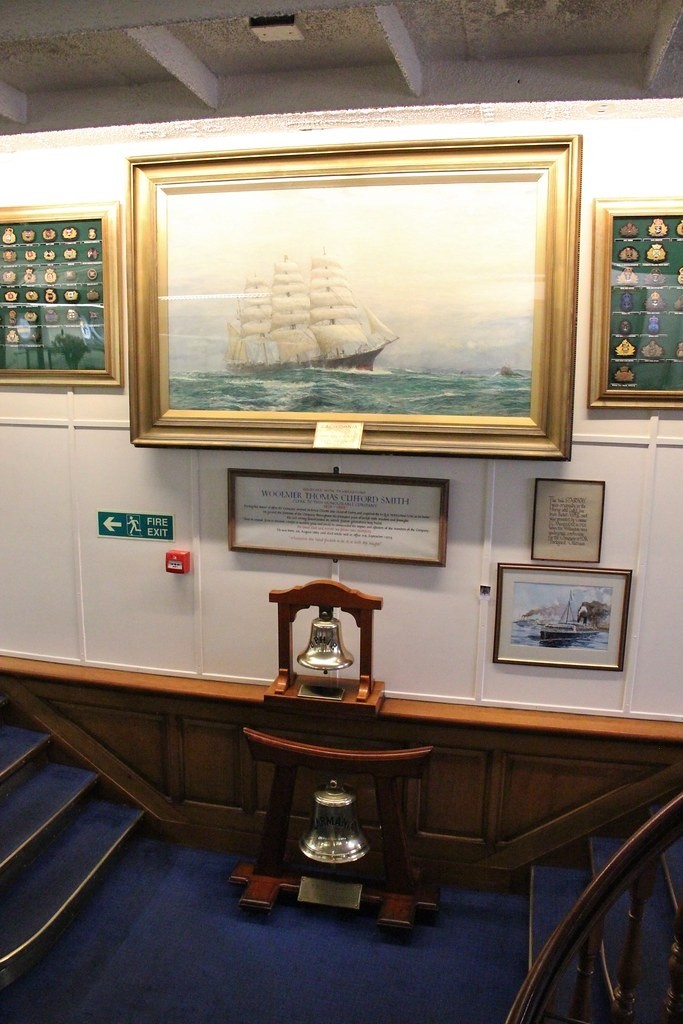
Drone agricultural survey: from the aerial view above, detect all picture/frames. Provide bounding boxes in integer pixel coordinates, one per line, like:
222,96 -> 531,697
127,132 -> 586,463
586,197 -> 683,410
0,199 -> 125,389
491,561 -> 632,672
531,477 -> 606,563
